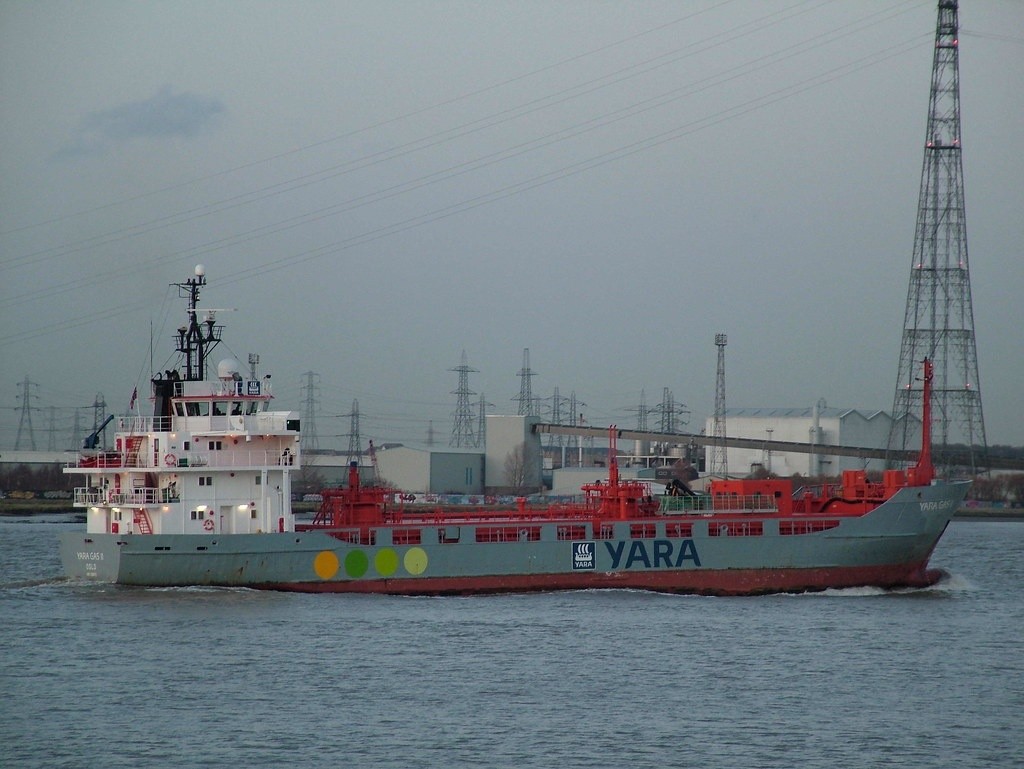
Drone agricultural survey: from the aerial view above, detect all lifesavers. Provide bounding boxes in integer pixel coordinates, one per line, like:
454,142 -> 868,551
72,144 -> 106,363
202,518 -> 215,531
164,454 -> 177,466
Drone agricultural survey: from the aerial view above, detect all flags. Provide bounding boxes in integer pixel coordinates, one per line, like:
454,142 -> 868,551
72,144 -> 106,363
129,388 -> 138,410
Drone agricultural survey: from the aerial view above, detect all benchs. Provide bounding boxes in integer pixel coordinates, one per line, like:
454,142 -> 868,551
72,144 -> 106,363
188,456 -> 208,466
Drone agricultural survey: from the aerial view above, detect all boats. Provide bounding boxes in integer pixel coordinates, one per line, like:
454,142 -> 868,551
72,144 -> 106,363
62,263 -> 973,598
